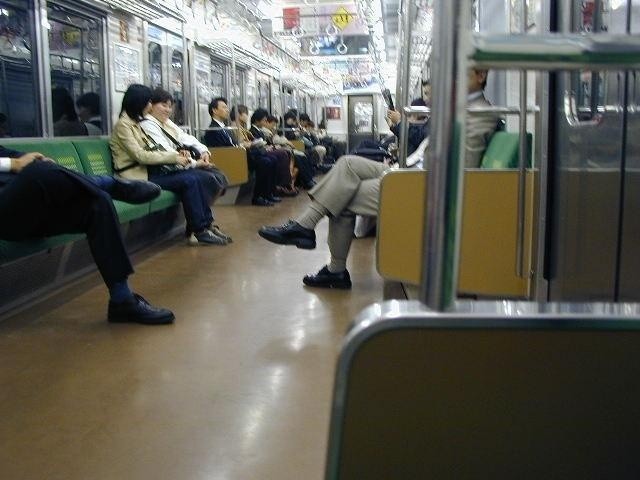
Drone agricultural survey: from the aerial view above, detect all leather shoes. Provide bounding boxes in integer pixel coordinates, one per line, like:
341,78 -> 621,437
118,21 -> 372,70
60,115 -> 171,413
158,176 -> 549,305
107,174 -> 162,205
258,219 -> 317,249
303,265 -> 352,288
185,228 -> 228,244
108,294 -> 175,324
251,155 -> 336,205
208,224 -> 233,242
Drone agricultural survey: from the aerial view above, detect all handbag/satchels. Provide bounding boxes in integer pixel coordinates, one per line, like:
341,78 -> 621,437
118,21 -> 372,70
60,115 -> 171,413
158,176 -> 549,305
147,143 -> 179,174
176,141 -> 201,161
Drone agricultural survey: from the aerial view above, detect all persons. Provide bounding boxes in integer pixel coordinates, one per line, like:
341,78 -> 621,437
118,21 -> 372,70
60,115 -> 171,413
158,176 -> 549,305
52,87 -> 103,137
258,69 -> 498,288
204,96 -> 336,207
0,147 -> 174,323
109,84 -> 233,245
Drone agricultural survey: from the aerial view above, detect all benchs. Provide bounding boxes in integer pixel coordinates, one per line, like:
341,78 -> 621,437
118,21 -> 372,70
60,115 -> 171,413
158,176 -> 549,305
386,128 -> 535,170
0,136 -> 203,237
